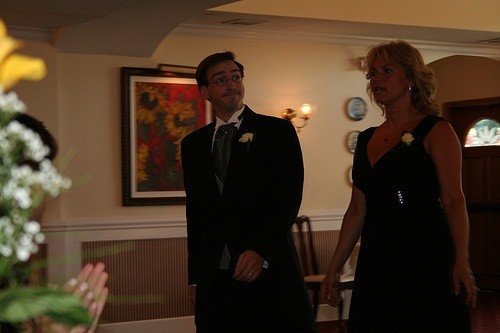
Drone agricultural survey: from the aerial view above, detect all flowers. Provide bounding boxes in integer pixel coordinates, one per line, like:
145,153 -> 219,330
0,19 -> 104,333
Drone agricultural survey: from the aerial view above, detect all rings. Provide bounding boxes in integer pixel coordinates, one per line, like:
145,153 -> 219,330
248,272 -> 253,276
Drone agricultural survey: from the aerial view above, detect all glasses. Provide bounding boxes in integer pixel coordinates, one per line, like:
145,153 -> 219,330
208,73 -> 243,87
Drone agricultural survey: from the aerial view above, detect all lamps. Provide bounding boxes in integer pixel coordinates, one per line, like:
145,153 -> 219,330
281,103 -> 312,134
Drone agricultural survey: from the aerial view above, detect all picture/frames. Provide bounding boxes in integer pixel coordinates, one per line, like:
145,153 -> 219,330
120,66 -> 216,207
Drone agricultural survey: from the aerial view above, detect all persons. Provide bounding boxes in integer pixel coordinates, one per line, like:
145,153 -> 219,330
0,112 -> 109,333
182,51 -> 316,333
319,41 -> 477,333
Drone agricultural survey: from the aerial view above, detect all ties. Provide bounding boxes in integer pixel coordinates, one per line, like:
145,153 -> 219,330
212,123 -> 235,193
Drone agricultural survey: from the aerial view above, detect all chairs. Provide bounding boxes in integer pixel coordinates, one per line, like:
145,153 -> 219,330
290,215 -> 354,333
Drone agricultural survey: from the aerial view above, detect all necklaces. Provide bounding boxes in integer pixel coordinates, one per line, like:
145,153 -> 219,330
384,128 -> 390,141
